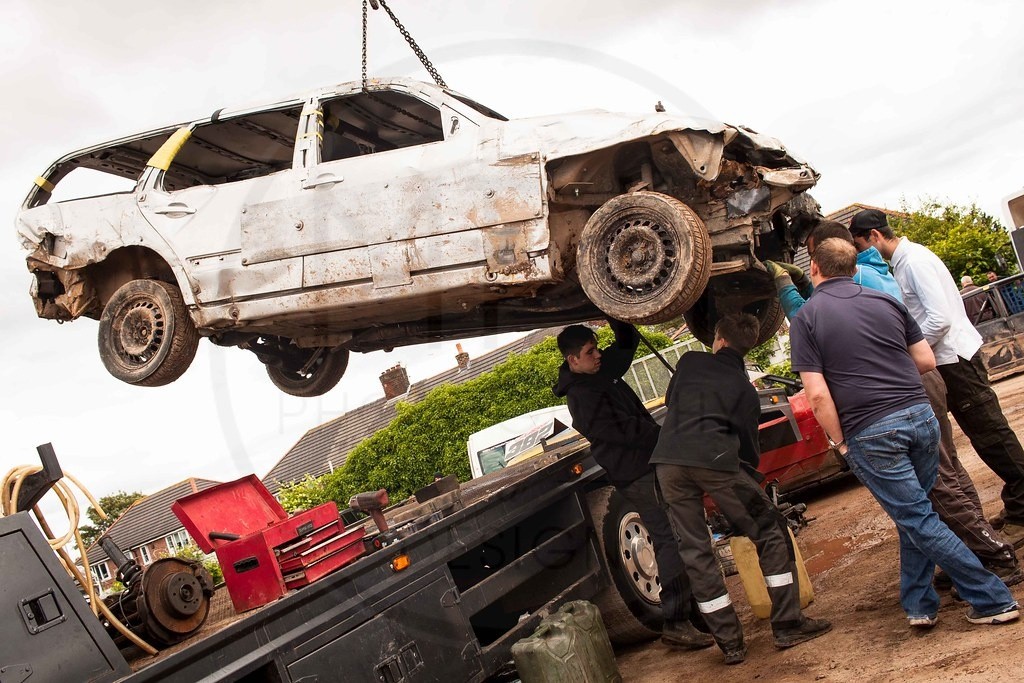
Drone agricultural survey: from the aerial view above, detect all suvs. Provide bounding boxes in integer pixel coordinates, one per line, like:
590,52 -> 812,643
14,76 -> 821,396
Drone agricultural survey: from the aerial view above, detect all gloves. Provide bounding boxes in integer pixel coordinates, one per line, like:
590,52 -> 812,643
776,260 -> 805,281
763,259 -> 789,280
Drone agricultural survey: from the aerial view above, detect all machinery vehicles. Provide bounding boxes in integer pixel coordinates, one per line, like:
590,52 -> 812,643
0,404 -> 812,683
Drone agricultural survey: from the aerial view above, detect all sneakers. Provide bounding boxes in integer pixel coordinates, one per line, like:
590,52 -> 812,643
965,603 -> 1019,625
909,615 -> 939,626
662,621 -> 714,647
723,646 -> 746,664
773,615 -> 833,647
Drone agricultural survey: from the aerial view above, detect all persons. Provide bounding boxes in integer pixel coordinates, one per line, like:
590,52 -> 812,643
849,209 -> 1023,531
763,223 -> 1015,649
788,237 -> 1023,625
553,317 -> 715,655
648,310 -> 834,665
981,271 -> 1021,319
959,274 -> 997,325
433,473 -> 443,483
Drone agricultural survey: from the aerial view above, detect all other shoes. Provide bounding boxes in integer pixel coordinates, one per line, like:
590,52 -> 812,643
996,518 -> 1024,550
951,561 -> 1024,602
989,508 -> 1006,529
933,569 -> 953,589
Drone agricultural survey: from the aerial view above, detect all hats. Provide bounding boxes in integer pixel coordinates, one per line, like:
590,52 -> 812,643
849,208 -> 888,234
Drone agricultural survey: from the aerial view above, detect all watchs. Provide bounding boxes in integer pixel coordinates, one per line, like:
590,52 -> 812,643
826,439 -> 846,450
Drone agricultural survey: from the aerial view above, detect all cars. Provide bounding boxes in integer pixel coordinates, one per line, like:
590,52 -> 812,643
702,373 -> 858,521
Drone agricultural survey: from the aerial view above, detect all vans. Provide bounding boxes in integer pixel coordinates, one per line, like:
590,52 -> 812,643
466,405 -> 575,483
